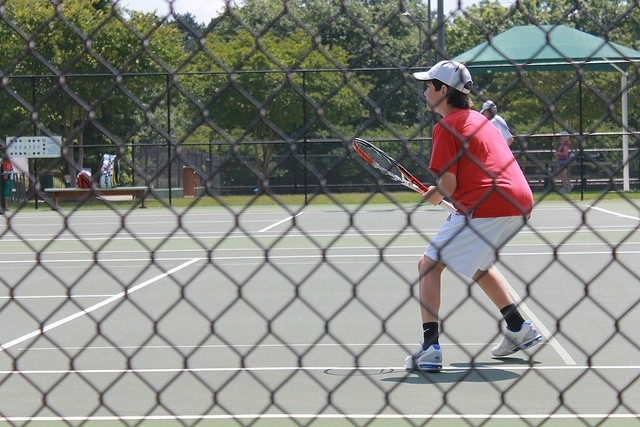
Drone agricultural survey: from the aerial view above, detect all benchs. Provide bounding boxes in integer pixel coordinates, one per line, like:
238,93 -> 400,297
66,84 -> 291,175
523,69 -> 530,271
44,186 -> 150,210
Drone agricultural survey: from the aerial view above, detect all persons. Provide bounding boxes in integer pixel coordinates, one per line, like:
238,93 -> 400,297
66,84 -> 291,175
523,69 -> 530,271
555,130 -> 574,193
404,57 -> 543,371
479,99 -> 514,147
2,149 -> 12,211
98,151 -> 120,189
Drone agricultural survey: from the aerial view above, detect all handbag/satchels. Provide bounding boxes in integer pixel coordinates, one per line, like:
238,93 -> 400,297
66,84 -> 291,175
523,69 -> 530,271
76,171 -> 92,189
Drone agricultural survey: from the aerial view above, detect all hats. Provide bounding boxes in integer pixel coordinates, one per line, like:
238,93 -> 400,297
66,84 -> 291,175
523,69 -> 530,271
413,59 -> 473,94
480,100 -> 498,115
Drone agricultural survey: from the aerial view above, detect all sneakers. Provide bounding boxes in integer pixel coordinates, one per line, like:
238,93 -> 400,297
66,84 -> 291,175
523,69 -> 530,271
405,343 -> 442,372
567,185 -> 574,191
559,188 -> 568,193
492,321 -> 542,356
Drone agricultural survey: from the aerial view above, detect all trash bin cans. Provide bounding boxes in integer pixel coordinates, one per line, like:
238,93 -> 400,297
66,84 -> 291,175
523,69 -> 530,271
183,166 -> 197,197
